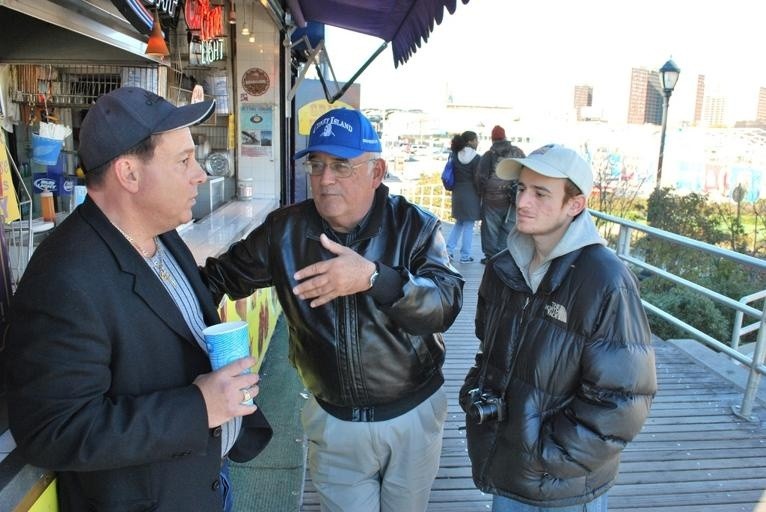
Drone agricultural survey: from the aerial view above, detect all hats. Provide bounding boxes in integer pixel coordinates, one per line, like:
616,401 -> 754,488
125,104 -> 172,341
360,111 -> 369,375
495,144 -> 593,197
79,88 -> 218,172
294,107 -> 381,161
491,125 -> 505,141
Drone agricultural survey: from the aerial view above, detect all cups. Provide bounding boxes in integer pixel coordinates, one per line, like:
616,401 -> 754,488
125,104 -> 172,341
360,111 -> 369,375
236,185 -> 252,200
199,319 -> 255,409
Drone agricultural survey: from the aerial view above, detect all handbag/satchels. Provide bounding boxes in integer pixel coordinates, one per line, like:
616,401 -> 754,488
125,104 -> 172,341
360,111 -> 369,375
441,161 -> 455,191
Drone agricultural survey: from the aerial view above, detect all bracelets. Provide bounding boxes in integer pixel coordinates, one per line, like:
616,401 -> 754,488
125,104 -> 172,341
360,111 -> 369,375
369,263 -> 379,288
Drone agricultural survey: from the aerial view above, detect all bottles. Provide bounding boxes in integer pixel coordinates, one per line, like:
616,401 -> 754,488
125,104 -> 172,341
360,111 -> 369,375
39,185 -> 56,226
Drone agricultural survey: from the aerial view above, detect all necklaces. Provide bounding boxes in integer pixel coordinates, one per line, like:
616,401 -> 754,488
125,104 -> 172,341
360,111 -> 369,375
112,224 -> 177,287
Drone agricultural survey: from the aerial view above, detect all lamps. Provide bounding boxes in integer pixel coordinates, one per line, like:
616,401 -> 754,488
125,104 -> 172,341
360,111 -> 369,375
249,1 -> 256,42
241,0 -> 249,35
229,0 -> 237,22
145,0 -> 169,56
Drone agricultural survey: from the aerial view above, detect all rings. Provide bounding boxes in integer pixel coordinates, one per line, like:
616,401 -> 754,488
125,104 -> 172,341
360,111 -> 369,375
242,388 -> 251,402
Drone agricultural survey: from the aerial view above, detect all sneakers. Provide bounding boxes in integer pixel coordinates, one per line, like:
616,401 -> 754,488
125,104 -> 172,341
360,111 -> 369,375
459,257 -> 473,263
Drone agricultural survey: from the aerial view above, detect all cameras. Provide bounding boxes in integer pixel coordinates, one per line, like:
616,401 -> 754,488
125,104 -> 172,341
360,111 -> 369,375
464,387 -> 505,426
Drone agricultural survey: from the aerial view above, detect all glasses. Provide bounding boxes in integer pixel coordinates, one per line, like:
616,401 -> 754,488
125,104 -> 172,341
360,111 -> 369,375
301,158 -> 379,179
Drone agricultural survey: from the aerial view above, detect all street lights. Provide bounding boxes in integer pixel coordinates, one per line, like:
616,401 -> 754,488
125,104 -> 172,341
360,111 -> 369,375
656,58 -> 681,190
732,183 -> 745,225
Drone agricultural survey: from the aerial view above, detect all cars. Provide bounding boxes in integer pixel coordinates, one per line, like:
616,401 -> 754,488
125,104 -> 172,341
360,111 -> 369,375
393,139 -> 451,157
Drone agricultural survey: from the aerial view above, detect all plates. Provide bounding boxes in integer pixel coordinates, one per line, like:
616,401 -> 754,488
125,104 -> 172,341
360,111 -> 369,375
30,221 -> 55,233
205,152 -> 230,177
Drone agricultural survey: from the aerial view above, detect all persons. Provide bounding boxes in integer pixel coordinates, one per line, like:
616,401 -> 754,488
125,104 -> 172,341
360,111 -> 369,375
458,145 -> 657,511
197,107 -> 466,512
446,131 -> 483,263
0,86 -> 273,512
474,125 -> 526,265
